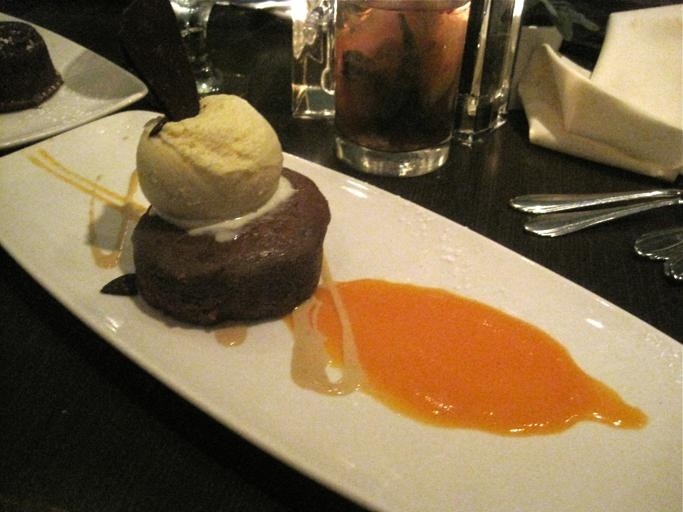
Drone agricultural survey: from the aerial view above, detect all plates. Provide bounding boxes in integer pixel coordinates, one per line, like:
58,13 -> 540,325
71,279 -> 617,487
0,109 -> 683,512
0,12 -> 148,157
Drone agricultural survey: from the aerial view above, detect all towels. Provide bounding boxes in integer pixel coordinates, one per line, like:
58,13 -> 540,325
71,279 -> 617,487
513,2 -> 682,182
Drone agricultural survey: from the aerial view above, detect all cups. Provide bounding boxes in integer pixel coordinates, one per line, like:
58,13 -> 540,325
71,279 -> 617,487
333,0 -> 473,176
449,0 -> 525,148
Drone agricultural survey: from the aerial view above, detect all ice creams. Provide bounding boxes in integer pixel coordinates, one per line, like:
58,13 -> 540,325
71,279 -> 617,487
101,0 -> 331,326
0,22 -> 64,113
